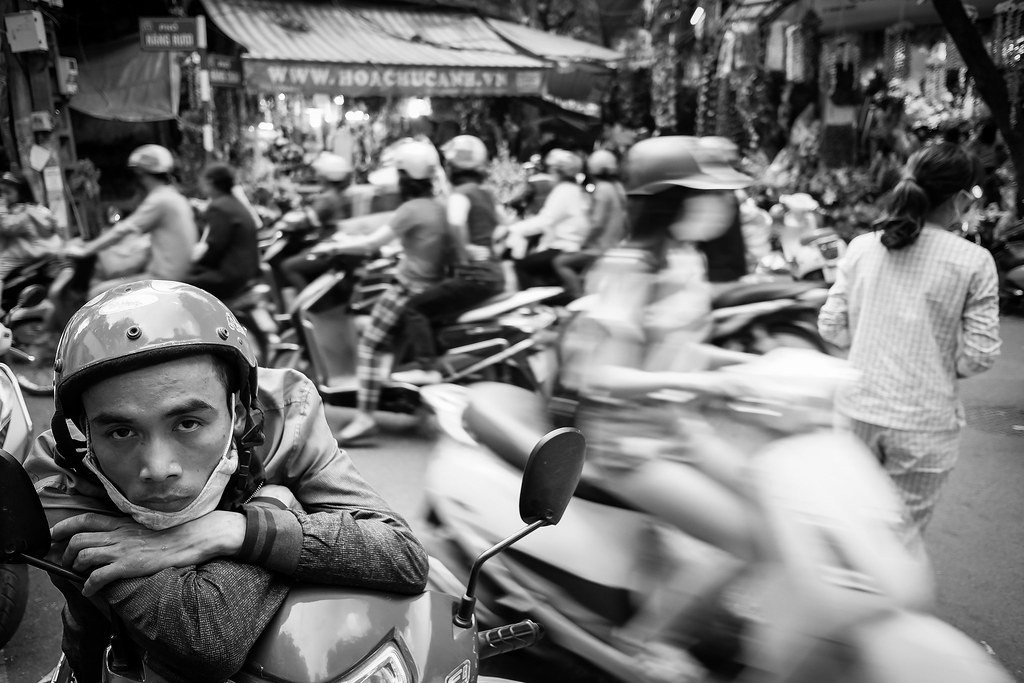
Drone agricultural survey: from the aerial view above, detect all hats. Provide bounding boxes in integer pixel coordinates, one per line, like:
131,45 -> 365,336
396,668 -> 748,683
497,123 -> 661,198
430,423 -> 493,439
545,148 -> 583,177
586,150 -> 618,175
623,137 -> 755,195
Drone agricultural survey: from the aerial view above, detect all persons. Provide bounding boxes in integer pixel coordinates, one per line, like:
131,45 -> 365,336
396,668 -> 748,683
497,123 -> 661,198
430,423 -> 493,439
817,144 -> 1000,538
0,127 -> 784,665
23,279 -> 429,683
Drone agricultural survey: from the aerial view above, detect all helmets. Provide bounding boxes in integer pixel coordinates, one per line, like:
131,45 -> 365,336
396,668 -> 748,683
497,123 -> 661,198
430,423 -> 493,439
51,278 -> 266,417
378,138 -> 438,180
439,135 -> 488,170
127,144 -> 174,173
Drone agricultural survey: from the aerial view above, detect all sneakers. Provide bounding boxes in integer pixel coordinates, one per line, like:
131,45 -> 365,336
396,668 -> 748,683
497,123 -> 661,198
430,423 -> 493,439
609,627 -> 709,683
339,418 -> 375,439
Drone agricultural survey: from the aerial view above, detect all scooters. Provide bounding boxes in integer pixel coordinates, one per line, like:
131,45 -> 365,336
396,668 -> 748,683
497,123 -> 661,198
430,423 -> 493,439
414,288 -> 1018,683
0,429 -> 585,683
1,221 -> 845,365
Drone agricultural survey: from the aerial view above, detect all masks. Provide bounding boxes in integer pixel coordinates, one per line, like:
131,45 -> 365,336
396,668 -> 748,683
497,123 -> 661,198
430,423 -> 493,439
80,387 -> 236,528
952,189 -> 980,228
665,196 -> 735,244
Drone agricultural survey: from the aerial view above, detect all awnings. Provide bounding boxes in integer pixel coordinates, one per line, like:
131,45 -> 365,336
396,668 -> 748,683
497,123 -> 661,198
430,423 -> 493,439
207,0 -> 615,97
